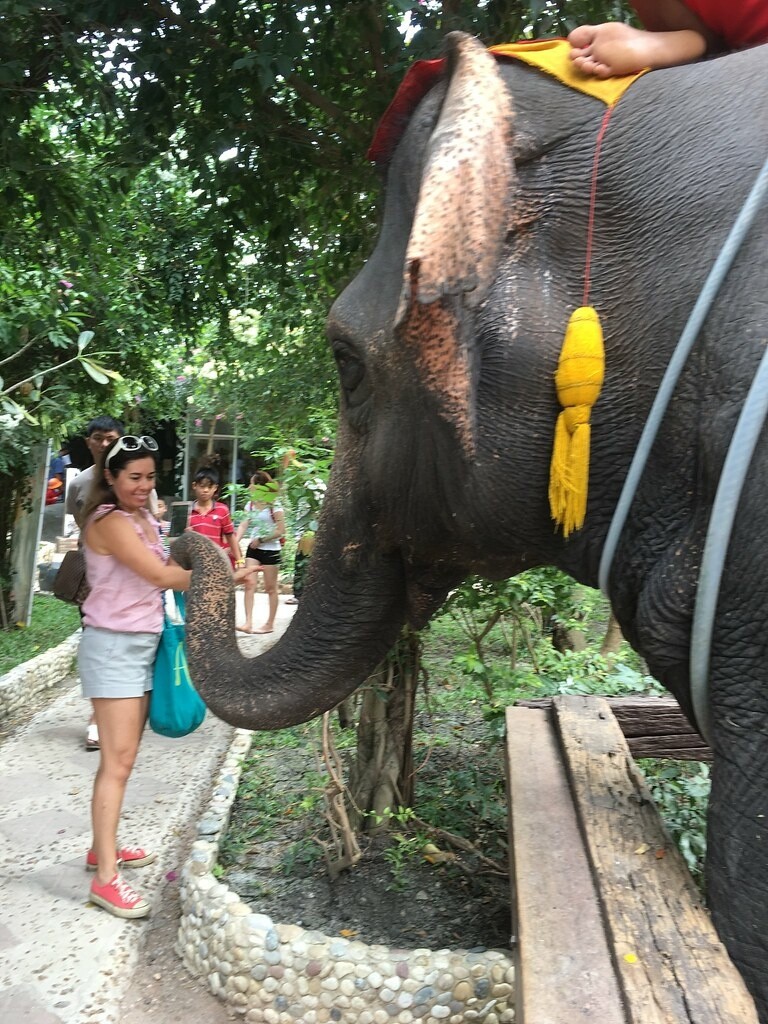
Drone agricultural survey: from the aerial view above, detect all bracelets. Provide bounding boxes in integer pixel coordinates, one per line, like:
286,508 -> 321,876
258,537 -> 265,544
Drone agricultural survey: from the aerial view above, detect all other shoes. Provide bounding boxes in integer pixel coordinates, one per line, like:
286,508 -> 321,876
285,597 -> 299,604
236,625 -> 252,634
253,627 -> 274,633
85,722 -> 101,749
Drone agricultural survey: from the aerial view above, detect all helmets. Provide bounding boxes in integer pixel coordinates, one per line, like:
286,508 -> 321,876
47,478 -> 62,489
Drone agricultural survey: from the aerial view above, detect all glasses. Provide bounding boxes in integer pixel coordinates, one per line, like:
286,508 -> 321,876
105,435 -> 158,468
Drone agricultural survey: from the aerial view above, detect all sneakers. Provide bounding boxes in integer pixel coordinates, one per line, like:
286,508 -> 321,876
89,871 -> 151,919
86,842 -> 153,870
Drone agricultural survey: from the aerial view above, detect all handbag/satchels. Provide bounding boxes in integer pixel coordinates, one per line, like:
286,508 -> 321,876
148,587 -> 207,738
53,550 -> 91,605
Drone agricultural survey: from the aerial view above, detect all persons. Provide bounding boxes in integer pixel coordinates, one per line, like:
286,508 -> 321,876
156,498 -> 173,557
236,471 -> 286,634
568,0 -> 768,78
78,436 -> 267,919
45,442 -> 71,506
279,449 -> 327,604
65,421 -> 158,749
189,470 -> 240,573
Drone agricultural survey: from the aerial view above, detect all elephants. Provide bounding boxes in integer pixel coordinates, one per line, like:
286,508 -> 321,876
179,31 -> 768,1024
